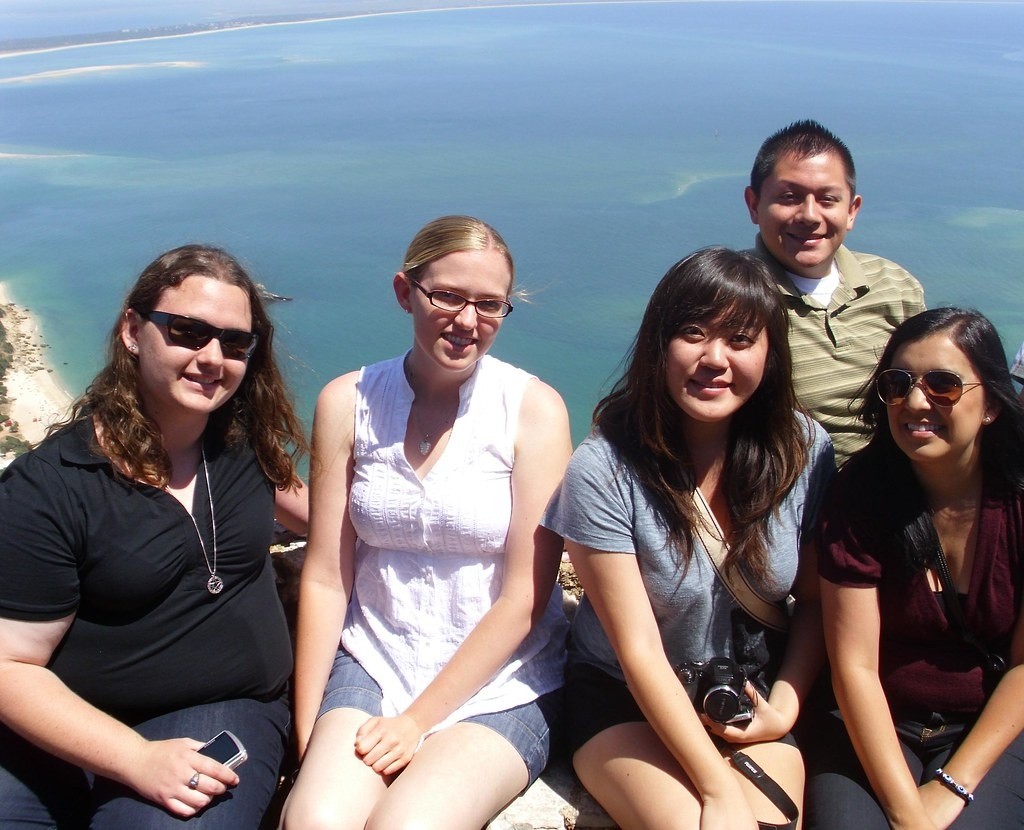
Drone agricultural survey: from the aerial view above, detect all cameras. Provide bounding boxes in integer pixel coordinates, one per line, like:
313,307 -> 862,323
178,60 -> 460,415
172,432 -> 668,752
673,656 -> 762,724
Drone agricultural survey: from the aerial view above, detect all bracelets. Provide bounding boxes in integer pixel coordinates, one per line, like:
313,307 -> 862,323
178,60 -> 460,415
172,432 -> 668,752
934,768 -> 973,804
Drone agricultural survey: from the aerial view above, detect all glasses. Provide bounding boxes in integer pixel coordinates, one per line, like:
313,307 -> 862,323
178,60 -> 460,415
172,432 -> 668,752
406,273 -> 514,318
146,308 -> 259,361
876,368 -> 984,408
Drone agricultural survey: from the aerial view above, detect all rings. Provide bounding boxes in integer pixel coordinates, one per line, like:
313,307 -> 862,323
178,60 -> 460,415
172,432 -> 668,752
189,771 -> 200,787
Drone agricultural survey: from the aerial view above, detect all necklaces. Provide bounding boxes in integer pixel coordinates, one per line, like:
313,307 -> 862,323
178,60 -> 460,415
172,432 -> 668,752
405,364 -> 458,455
163,442 -> 224,594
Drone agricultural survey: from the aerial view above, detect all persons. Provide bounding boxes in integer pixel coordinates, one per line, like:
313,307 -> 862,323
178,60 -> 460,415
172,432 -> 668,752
283,216 -> 572,829
0,244 -> 323,830
540,246 -> 836,829
819,307 -> 1023,829
737,119 -> 926,472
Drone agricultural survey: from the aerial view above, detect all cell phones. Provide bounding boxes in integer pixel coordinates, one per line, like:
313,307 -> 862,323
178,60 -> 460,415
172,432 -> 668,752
725,712 -> 752,724
197,729 -> 248,771
